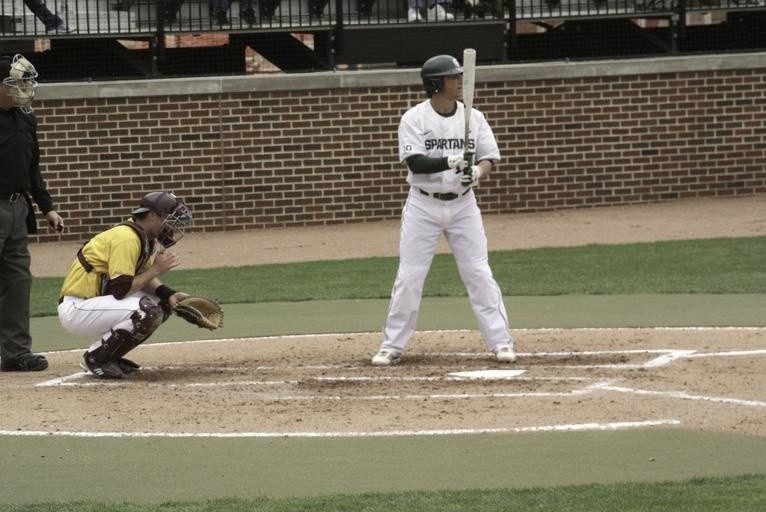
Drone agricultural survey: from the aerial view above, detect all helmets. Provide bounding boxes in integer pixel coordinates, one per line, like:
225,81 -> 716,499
420,53 -> 465,78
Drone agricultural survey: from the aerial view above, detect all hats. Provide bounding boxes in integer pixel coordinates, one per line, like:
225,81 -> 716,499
0,54 -> 38,84
130,188 -> 187,221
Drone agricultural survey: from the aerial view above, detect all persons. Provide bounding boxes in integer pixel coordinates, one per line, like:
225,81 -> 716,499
371,54 -> 517,367
408,0 -> 454,23
109,0 -> 376,26
2,53 -> 64,371
58,191 -> 195,379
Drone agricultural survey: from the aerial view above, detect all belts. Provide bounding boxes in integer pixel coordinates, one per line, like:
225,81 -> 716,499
57,294 -> 87,305
418,186 -> 472,201
0,191 -> 27,204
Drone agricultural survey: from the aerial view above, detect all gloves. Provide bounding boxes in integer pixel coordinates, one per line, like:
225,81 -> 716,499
448,150 -> 470,172
455,164 -> 482,190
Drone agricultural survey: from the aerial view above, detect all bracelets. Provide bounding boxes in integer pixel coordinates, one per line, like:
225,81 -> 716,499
155,284 -> 177,300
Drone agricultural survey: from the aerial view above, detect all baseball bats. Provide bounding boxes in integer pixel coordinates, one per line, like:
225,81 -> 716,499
463,49 -> 476,186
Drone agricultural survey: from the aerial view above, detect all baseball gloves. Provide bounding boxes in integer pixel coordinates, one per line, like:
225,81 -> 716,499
175,296 -> 223,330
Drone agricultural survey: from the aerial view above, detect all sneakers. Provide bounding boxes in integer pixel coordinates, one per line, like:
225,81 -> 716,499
109,356 -> 133,374
369,346 -> 403,367
0,351 -> 49,372
494,345 -> 518,364
78,350 -> 128,380
